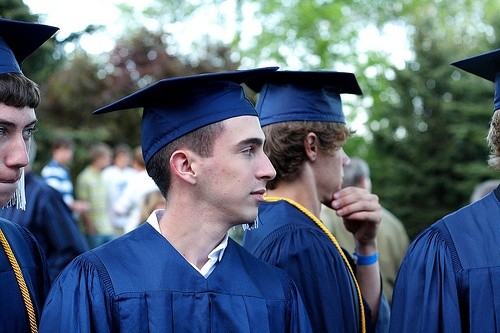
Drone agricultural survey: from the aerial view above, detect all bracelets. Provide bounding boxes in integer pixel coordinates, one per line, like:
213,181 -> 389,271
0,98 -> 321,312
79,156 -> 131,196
353,249 -> 378,265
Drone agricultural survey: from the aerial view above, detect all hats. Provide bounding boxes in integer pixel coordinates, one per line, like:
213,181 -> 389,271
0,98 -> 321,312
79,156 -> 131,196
243,69 -> 363,126
93,66 -> 280,167
449,48 -> 500,111
0,17 -> 60,210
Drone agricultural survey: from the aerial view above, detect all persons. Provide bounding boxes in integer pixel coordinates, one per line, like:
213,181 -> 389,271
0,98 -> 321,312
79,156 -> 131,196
75,143 -> 111,248
114,145 -> 166,233
43,67 -> 312,333
104,143 -> 139,230
390,49 -> 500,333
41,132 -> 90,219
321,157 -> 410,306
0,140 -> 90,285
245,70 -> 390,333
0,19 -> 59,332
